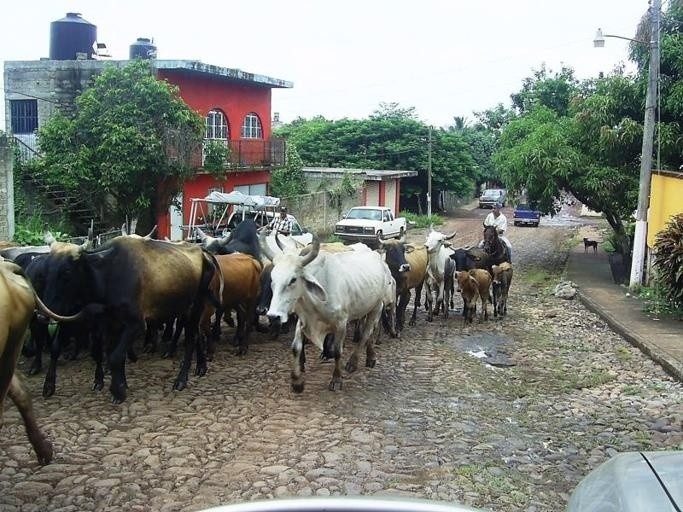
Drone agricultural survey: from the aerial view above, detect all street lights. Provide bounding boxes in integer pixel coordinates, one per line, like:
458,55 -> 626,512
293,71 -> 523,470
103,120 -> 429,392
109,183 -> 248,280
593,0 -> 661,293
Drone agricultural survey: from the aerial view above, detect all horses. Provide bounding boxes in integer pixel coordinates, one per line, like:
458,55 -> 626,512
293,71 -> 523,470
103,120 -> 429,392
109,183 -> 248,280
483,221 -> 508,305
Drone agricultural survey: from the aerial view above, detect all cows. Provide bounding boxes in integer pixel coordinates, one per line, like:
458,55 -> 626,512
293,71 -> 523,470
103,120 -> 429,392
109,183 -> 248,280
256,228 -> 396,394
376,222 -> 488,331
491,262 -> 513,316
0,254 -> 105,467
3,218 -> 256,375
27,234 -> 226,406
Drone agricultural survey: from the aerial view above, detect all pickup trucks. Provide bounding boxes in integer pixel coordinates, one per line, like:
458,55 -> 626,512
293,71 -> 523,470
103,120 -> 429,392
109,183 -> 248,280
333,206 -> 407,244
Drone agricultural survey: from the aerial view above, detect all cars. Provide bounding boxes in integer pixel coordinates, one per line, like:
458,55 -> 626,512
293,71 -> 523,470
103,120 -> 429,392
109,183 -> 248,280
513,202 -> 540,227
222,210 -> 308,238
479,189 -> 505,208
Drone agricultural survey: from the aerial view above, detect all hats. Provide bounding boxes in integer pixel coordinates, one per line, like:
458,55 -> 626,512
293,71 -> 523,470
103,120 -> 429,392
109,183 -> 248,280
489,201 -> 503,208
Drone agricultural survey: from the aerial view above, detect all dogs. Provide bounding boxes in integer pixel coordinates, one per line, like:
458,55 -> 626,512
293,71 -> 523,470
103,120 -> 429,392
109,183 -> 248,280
584,238 -> 599,251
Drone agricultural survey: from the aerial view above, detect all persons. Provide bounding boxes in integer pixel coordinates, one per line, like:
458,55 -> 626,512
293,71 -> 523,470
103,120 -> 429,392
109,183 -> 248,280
477,202 -> 513,265
265,206 -> 291,236
